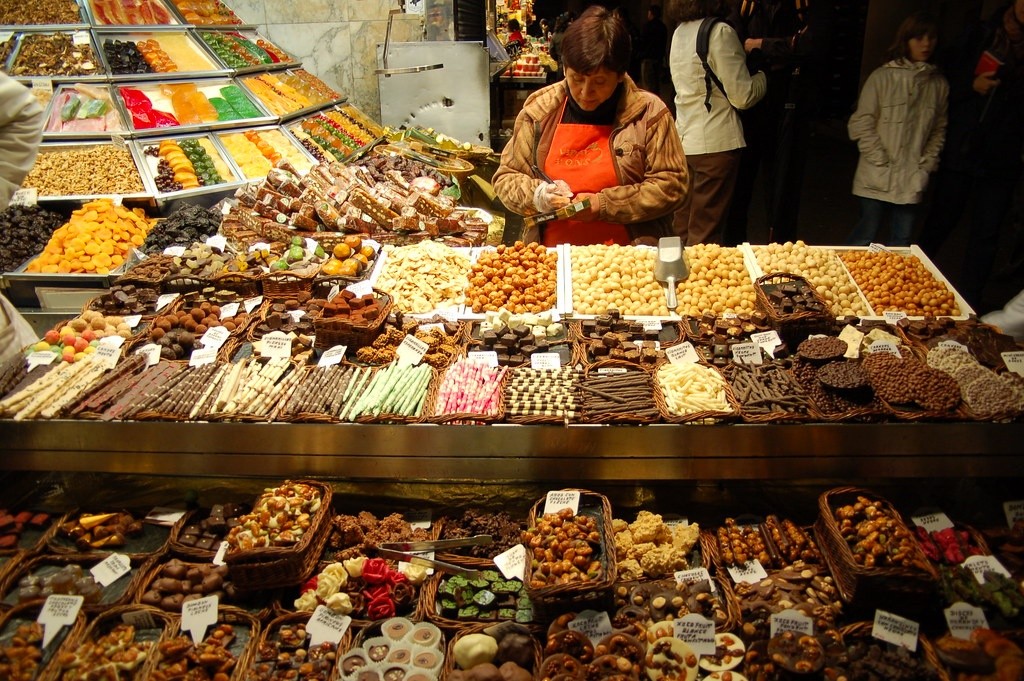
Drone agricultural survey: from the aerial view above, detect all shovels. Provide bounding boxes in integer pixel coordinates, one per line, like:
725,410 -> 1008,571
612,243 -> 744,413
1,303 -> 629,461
654,236 -> 690,309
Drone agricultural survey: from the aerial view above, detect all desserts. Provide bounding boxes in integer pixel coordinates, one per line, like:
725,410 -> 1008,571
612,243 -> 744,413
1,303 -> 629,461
0,0 -> 385,197
0,126 -> 1024,422
0,481 -> 1024,681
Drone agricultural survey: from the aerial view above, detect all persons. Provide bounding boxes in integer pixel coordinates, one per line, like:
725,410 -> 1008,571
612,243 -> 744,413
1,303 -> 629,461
670,0 -> 766,244
507,19 -> 524,45
638,5 -> 668,92
549,11 -> 576,82
941,0 -> 1023,316
846,11 -> 953,246
492,5 -> 689,246
714,1 -> 833,248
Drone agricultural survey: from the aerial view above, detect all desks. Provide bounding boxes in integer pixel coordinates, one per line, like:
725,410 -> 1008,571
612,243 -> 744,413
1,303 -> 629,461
0,415 -> 1024,481
500,72 -> 547,88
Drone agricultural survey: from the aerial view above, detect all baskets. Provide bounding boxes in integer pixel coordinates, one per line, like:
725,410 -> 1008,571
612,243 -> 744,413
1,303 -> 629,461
0,229 -> 1024,681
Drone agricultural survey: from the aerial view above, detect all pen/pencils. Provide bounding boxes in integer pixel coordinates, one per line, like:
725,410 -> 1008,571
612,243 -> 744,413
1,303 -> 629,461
532,165 -> 558,187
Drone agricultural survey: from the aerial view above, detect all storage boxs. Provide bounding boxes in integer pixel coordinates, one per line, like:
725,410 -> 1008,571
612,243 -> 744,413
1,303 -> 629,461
504,90 -> 535,116
